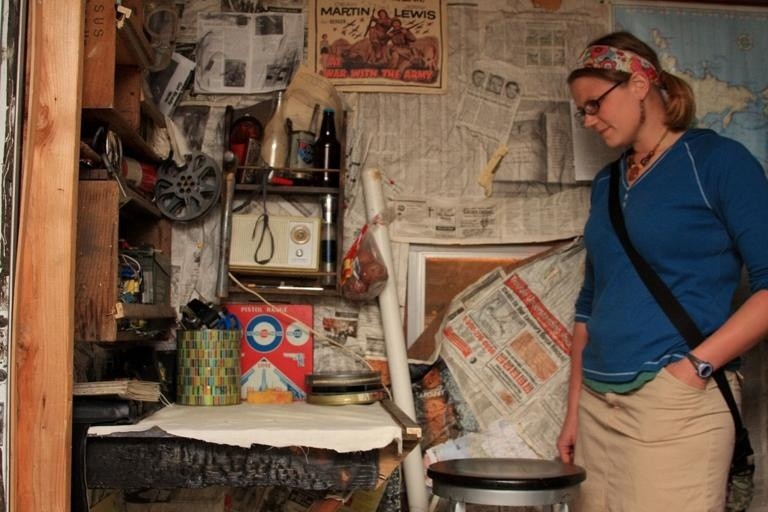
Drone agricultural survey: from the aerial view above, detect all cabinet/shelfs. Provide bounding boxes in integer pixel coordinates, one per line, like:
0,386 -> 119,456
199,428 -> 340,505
214,153 -> 345,301
83,3 -> 164,157
77,178 -> 178,343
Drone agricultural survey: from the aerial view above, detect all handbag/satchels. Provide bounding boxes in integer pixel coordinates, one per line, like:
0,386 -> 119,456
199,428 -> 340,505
723,428 -> 755,512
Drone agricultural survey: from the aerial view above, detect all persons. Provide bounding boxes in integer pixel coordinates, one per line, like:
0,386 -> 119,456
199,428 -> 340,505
319,33 -> 329,71
556,29 -> 768,510
369,10 -> 393,62
380,19 -> 419,71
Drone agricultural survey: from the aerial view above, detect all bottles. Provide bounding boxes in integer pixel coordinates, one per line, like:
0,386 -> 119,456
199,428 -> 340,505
320,194 -> 338,287
261,90 -> 338,185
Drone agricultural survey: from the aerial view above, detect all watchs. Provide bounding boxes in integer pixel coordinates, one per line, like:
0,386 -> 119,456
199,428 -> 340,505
686,351 -> 714,379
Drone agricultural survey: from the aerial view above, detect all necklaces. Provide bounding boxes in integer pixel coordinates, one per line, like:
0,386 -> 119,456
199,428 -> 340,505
625,127 -> 669,181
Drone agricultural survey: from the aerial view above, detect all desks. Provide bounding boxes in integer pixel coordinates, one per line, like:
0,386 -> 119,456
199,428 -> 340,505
84,399 -> 423,492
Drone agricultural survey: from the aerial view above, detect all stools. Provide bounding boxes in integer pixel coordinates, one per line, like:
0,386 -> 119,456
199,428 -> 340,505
426,457 -> 587,511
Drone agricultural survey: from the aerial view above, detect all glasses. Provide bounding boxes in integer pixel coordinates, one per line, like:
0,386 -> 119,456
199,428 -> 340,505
573,82 -> 622,123
